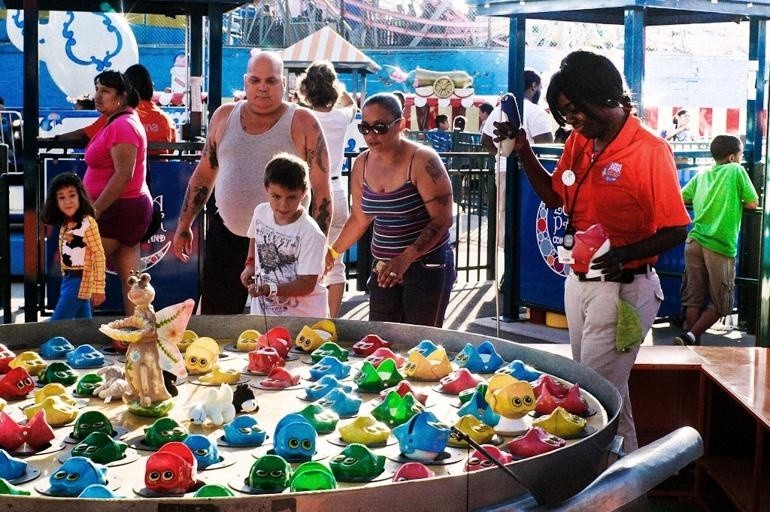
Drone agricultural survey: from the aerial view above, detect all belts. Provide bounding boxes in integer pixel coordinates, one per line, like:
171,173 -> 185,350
577,265 -> 652,286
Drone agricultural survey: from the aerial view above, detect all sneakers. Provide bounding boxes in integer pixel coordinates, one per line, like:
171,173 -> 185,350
673,332 -> 694,346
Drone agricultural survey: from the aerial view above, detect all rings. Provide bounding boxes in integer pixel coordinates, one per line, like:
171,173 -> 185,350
389,272 -> 398,279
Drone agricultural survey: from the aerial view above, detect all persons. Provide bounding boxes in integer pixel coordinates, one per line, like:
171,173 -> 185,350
314,90 -> 458,328
174,50 -> 335,316
504,50 -> 693,511
46,62 -> 177,162
39,171 -> 110,318
426,70 -> 561,169
297,57 -> 358,318
239,152 -> 334,316
671,130 -> 759,345
81,67 -> 156,315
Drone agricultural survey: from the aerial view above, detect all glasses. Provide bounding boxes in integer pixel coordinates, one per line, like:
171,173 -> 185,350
356,118 -> 401,134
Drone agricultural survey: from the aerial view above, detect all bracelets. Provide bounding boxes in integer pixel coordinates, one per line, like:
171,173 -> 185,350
263,281 -> 277,299
327,247 -> 344,261
245,256 -> 256,269
